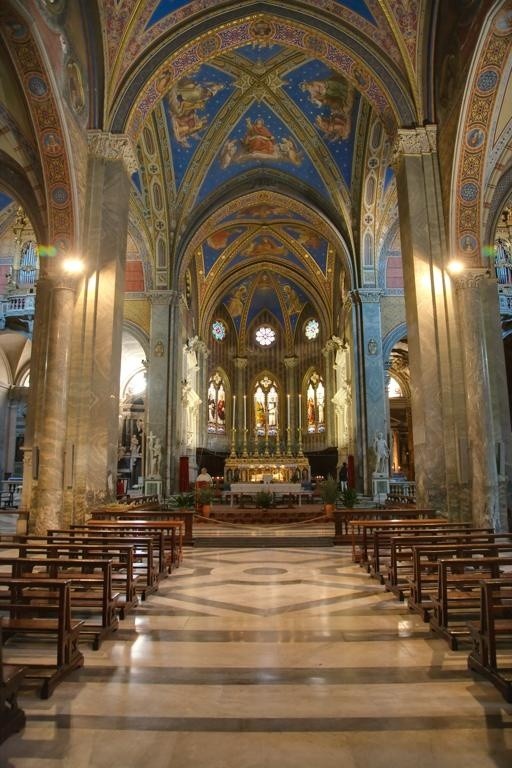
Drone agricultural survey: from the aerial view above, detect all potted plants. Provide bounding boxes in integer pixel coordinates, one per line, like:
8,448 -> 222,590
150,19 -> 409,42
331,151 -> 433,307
167,486 -> 214,519
317,474 -> 360,520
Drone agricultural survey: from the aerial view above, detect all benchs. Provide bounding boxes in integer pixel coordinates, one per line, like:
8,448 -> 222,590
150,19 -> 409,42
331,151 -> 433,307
350,520 -> 511,702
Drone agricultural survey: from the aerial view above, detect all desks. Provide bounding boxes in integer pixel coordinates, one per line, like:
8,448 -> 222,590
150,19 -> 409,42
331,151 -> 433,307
2,478 -> 25,509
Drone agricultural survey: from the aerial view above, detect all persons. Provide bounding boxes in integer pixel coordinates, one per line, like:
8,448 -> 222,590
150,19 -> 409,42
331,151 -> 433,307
372,432 -> 389,474
243,117 -> 276,154
129,434 -> 139,452
339,463 -> 349,494
150,438 -> 161,476
195,468 -> 213,485
174,79 -> 218,137
209,205 -> 320,316
300,79 -> 349,102
